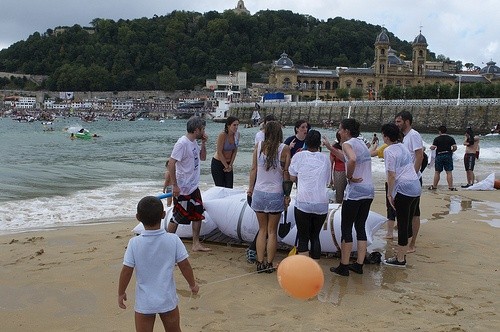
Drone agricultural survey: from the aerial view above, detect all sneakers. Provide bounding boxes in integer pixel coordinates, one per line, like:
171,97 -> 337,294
351,262 -> 363,274
330,263 -> 350,276
383,255 -> 407,267
247,248 -> 256,264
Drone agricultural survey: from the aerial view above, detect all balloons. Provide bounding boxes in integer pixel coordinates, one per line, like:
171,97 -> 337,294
275,255 -> 324,300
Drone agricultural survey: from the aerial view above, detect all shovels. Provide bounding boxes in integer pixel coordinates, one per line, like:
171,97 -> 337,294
278,196 -> 291,238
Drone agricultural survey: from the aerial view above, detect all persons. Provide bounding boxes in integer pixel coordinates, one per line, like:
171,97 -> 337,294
210,117 -> 240,189
321,118 -> 375,276
323,119 -> 334,129
489,125 -> 498,135
283,120 -> 322,197
381,122 -> 422,268
43,126 -> 99,139
243,116 -> 287,128
247,120 -> 291,274
287,130 -> 332,263
370,131 -> 405,239
371,133 -> 379,147
163,160 -> 174,207
461,128 -> 480,188
357,134 -> 371,149
254,114 -> 279,146
392,110 -> 424,253
118,196 -> 199,332
167,116 -> 212,251
0,106 -> 176,124
428,125 -> 458,191
329,130 -> 348,203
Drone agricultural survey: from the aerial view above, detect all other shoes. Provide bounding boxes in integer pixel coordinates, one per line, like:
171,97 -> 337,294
428,186 -> 437,190
257,260 -> 265,273
266,262 -> 275,273
449,188 -> 457,191
462,184 -> 473,188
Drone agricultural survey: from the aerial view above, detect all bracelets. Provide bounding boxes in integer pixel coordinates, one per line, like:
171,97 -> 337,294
346,175 -> 354,181
